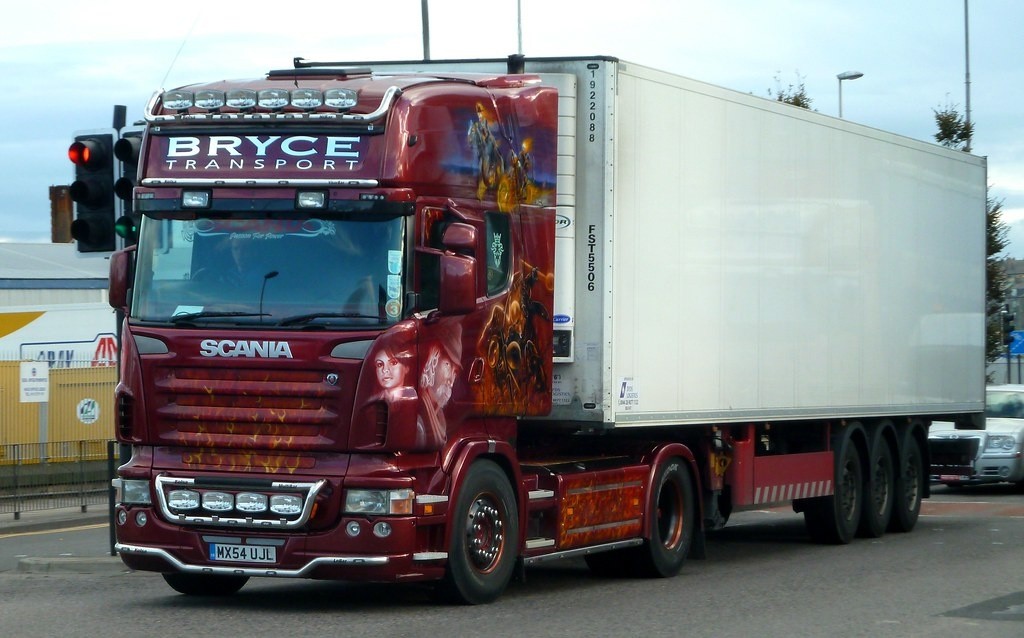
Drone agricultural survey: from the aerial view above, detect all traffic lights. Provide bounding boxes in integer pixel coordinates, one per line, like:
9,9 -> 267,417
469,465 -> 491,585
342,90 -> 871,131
113,130 -> 147,242
67,134 -> 118,254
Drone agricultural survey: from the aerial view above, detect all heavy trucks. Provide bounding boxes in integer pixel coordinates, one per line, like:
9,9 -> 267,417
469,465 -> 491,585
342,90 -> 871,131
103,55 -> 989,607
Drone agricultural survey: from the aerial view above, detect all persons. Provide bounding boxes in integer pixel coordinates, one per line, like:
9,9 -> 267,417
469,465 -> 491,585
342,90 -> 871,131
213,232 -> 271,303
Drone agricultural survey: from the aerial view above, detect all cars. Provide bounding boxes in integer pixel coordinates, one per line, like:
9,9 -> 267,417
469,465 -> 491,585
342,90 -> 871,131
940,383 -> 1024,490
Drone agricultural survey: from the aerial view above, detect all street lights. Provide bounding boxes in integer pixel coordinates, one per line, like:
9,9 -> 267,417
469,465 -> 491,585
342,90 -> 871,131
836,67 -> 864,117
